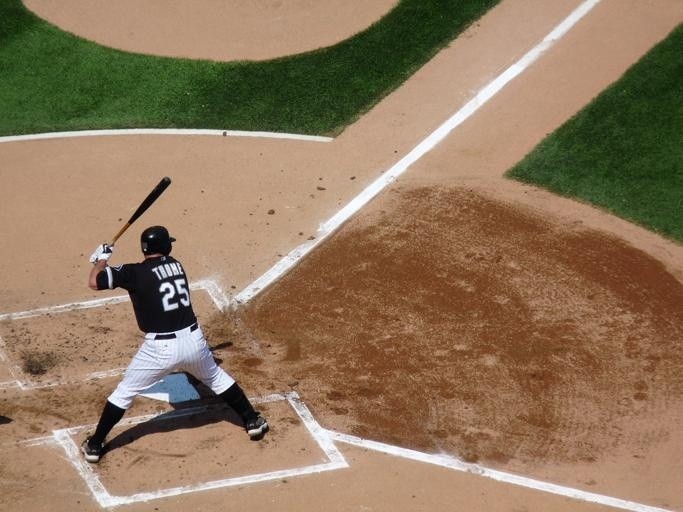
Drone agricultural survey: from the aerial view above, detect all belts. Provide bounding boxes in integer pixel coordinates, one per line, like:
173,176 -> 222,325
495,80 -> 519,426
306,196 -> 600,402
154,324 -> 198,340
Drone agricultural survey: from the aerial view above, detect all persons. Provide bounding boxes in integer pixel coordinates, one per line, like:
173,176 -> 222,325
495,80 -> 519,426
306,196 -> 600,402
78,225 -> 269,463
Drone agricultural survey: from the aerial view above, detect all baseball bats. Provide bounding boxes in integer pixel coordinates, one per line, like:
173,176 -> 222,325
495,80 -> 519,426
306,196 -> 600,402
110,176 -> 171,245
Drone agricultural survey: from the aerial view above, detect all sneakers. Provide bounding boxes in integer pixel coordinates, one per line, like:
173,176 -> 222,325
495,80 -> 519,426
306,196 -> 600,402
81,436 -> 101,463
246,416 -> 267,436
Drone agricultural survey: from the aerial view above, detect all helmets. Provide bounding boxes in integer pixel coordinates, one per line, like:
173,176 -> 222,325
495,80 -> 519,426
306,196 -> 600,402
141,226 -> 176,256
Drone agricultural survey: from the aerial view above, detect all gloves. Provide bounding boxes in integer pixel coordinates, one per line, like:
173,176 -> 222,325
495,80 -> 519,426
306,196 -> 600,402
89,243 -> 115,263
